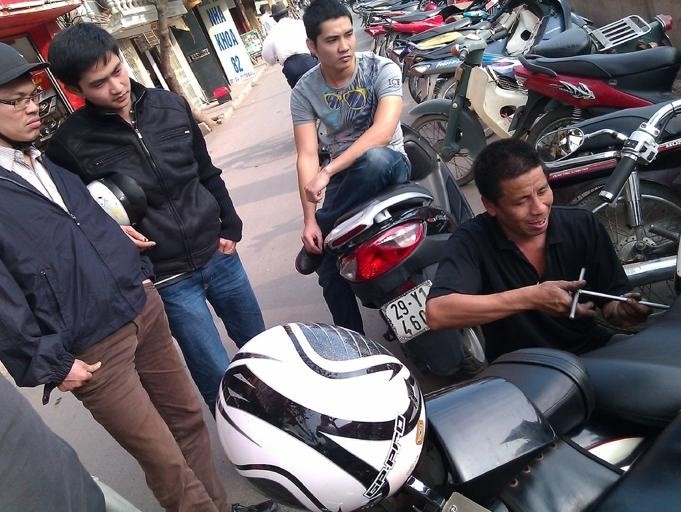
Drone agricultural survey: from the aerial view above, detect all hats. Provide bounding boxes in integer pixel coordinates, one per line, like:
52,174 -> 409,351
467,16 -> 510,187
268,1 -> 291,18
0,42 -> 51,86
86,172 -> 149,228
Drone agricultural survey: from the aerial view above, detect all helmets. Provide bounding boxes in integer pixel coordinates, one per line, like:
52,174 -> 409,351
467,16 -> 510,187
211,318 -> 430,512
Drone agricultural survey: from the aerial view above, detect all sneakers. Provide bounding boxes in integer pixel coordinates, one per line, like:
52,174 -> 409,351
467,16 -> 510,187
293,245 -> 325,276
232,499 -> 278,512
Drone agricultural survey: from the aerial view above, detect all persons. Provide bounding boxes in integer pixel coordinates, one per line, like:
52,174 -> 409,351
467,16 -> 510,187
0,369 -> 149,511
261,3 -> 322,88
293,1 -> 415,340
258,4 -> 277,36
424,137 -> 657,364
33,20 -> 268,420
0,40 -> 279,510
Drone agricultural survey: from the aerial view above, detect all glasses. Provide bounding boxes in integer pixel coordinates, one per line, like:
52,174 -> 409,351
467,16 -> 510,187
0,86 -> 45,112
